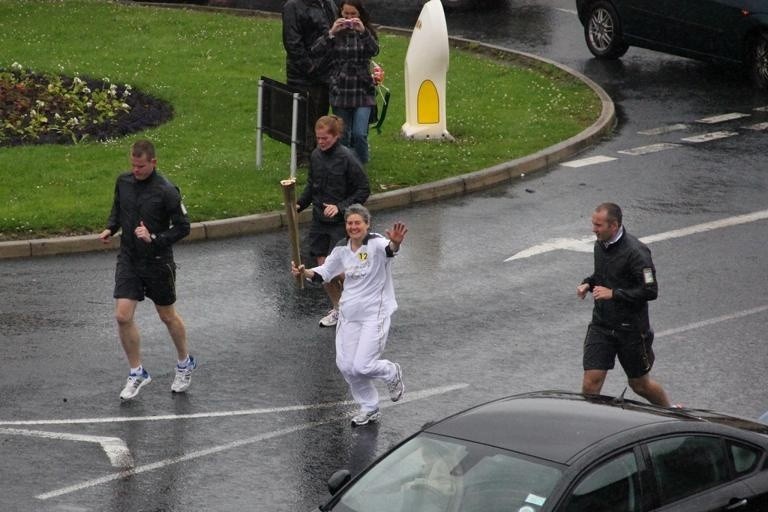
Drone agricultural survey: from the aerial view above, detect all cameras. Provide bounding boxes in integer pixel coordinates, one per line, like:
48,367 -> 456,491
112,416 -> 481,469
341,19 -> 354,30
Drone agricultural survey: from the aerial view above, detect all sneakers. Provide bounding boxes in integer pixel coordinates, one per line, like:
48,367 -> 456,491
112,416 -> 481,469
351,407 -> 382,427
170,354 -> 200,394
119,366 -> 152,403
318,307 -> 340,328
385,361 -> 404,402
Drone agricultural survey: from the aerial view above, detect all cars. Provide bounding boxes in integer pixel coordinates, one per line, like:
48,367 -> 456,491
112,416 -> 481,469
307,381 -> 766,510
569,0 -> 767,86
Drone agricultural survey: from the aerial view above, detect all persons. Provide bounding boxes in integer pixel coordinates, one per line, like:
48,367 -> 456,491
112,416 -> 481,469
281,114 -> 371,327
577,204 -> 683,408
310,0 -> 380,163
99,141 -> 197,400
283,0 -> 340,167
291,203 -> 409,425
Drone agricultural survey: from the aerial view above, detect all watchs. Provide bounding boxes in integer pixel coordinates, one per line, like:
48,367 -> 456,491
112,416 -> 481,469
150,232 -> 157,244
